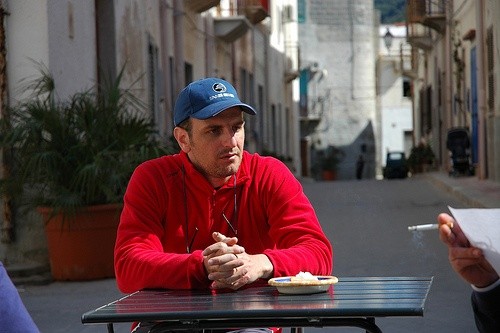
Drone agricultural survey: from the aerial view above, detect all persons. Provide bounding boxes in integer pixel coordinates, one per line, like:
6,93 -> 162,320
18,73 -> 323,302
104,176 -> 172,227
438,213 -> 500,333
114,77 -> 333,333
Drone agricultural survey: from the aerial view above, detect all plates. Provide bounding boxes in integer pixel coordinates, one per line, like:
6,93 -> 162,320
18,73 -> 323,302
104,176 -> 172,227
268,276 -> 339,294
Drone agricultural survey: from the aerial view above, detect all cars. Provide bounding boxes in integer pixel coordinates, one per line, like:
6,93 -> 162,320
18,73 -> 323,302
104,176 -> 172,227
384,151 -> 409,179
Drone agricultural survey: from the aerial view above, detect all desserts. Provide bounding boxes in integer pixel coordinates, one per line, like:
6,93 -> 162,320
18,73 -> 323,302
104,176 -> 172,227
290,271 -> 318,281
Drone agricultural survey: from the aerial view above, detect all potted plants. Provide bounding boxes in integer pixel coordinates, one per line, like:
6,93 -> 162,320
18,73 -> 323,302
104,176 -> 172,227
0,56 -> 175,281
321,156 -> 342,181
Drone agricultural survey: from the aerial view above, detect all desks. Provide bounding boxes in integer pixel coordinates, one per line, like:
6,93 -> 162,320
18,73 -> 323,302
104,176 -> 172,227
81,277 -> 435,333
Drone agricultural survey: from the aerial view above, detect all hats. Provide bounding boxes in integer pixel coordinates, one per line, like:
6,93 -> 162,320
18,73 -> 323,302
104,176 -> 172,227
175,77 -> 257,123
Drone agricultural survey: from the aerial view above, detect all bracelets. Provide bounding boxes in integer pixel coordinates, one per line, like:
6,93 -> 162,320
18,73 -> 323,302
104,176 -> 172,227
470,279 -> 500,292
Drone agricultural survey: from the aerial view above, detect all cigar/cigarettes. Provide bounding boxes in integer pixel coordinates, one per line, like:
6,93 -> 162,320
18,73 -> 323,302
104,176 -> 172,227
408,223 -> 453,231
